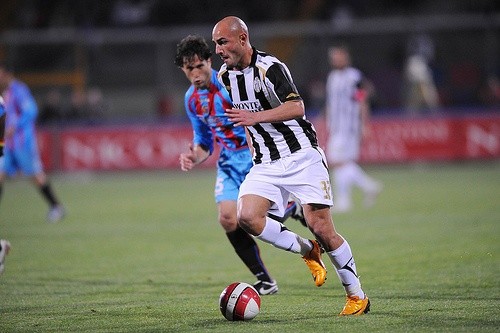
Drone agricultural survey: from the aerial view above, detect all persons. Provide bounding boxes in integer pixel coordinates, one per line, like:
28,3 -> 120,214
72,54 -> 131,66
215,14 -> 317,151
321,40 -> 380,214
212,16 -> 371,316
172,35 -> 306,295
0,67 -> 66,223
406,36 -> 442,112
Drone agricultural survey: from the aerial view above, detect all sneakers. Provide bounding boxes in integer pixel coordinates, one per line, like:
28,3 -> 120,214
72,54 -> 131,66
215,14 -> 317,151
301,239 -> 327,287
339,294 -> 371,316
252,280 -> 278,295
288,199 -> 306,227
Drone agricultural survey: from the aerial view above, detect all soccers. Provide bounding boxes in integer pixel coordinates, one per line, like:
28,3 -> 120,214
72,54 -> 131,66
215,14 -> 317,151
219,282 -> 261,322
